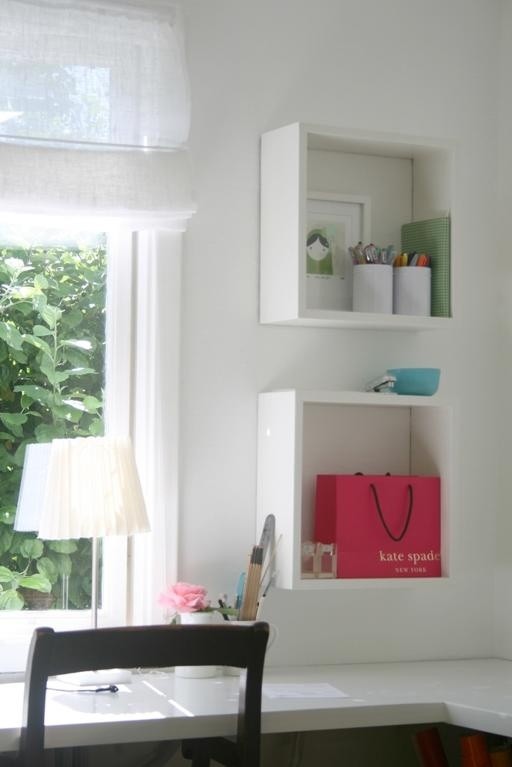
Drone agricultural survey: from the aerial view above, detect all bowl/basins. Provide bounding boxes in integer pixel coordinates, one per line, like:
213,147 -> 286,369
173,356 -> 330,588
386,366 -> 443,397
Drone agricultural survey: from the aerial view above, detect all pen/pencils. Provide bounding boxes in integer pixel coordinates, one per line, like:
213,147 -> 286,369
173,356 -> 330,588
217,599 -> 229,621
235,572 -> 246,620
349,242 -> 397,264
394,252 -> 431,266
256,594 -> 265,620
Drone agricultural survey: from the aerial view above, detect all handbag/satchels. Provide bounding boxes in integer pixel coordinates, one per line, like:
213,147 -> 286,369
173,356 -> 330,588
314,475 -> 440,578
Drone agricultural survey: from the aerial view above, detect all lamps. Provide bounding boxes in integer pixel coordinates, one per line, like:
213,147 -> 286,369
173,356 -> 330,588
35,434 -> 153,688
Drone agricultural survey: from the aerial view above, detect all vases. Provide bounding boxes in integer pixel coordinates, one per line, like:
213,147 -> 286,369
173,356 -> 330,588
174,608 -> 228,677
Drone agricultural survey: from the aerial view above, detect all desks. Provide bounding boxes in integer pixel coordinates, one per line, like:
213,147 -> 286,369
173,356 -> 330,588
0,659 -> 511,766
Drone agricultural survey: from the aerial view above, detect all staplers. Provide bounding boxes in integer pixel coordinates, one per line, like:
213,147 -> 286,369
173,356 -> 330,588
365,374 -> 398,395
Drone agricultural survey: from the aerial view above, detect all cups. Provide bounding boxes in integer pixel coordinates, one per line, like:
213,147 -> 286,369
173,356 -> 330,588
300,541 -> 339,579
222,619 -> 278,677
176,609 -> 216,680
351,263 -> 394,315
393,264 -> 434,317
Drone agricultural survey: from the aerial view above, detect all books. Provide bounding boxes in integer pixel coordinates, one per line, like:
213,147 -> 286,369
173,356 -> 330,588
400,217 -> 453,316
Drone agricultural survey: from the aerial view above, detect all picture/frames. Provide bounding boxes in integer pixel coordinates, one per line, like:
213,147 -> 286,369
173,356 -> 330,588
305,189 -> 373,317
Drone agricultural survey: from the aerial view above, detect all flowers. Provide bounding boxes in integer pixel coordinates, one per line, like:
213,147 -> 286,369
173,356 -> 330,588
164,567 -> 238,614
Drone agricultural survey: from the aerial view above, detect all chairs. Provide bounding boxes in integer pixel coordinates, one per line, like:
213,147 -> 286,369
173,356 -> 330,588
18,621 -> 270,765
